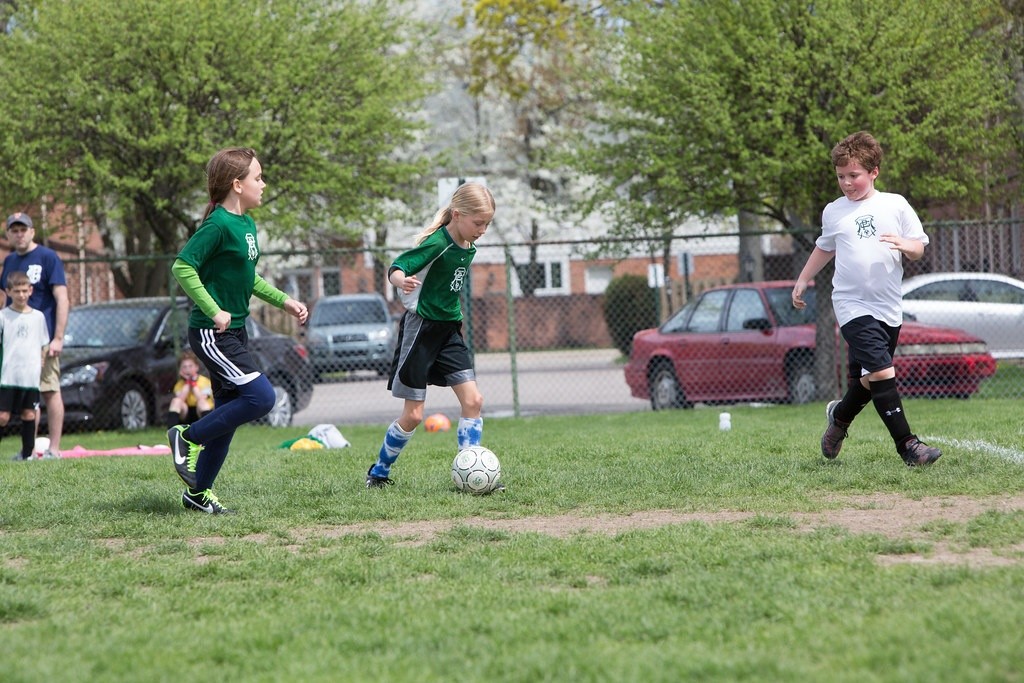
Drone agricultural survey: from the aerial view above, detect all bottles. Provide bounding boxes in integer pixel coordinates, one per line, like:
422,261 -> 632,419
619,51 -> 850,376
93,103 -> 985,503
718,412 -> 732,431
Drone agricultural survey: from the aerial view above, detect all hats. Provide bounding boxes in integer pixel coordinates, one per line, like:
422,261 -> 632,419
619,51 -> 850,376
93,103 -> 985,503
6,212 -> 33,229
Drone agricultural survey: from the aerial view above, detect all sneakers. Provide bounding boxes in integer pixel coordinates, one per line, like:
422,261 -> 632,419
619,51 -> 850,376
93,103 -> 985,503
365,463 -> 395,489
492,482 -> 506,491
168,424 -> 205,490
182,486 -> 240,517
896,433 -> 942,467
821,400 -> 850,459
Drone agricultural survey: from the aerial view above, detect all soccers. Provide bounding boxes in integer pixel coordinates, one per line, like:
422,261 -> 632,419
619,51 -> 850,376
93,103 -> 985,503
424,411 -> 452,434
450,445 -> 501,494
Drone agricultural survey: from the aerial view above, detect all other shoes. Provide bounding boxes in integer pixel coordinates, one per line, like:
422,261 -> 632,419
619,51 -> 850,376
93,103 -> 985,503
39,450 -> 62,461
11,449 -> 33,462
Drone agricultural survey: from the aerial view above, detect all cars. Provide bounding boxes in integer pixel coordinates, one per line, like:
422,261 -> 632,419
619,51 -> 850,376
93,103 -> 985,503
623,279 -> 1000,411
303,293 -> 401,385
900,271 -> 1024,361
0,296 -> 316,434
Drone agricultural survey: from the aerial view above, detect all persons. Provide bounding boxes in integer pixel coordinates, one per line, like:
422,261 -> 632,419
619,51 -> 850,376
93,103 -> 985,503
364,182 -> 506,493
0,270 -> 51,459
165,148 -> 309,515
0,212 -> 70,460
166,351 -> 215,429
791,130 -> 942,468
